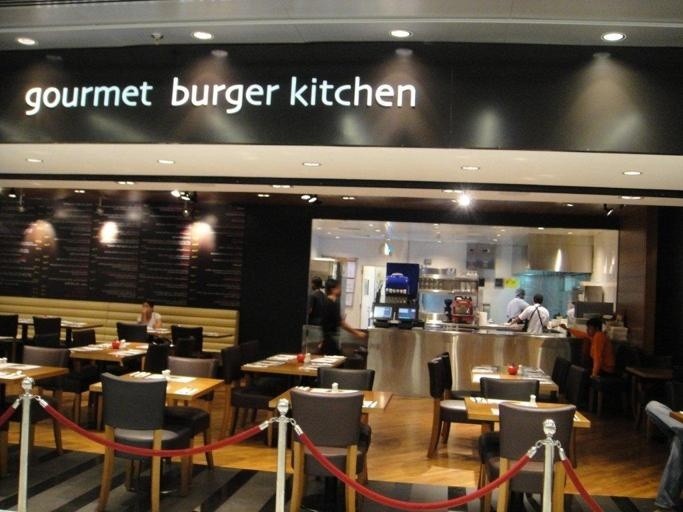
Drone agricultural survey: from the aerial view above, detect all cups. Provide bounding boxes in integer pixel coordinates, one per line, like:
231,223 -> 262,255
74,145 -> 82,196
432,312 -> 438,321
478,311 -> 488,326
459,281 -> 477,292
506,368 -> 518,376
112,341 -> 119,349
296,355 -> 305,363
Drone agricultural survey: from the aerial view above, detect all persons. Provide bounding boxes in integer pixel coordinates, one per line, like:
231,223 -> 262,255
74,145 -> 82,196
560,317 -> 617,409
509,293 -> 550,335
505,288 -> 530,323
306,276 -> 327,326
137,299 -> 162,342
318,278 -> 367,356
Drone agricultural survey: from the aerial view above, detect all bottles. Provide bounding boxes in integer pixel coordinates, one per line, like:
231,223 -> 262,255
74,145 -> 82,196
385,288 -> 408,305
305,352 -> 311,364
420,278 -> 443,290
162,369 -> 171,383
517,365 -> 524,376
331,382 -> 339,393
529,394 -> 538,407
119,340 -> 126,350
0,357 -> 9,371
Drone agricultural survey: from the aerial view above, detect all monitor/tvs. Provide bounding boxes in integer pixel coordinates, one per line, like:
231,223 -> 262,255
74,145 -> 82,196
372,304 -> 394,320
395,304 -> 418,321
